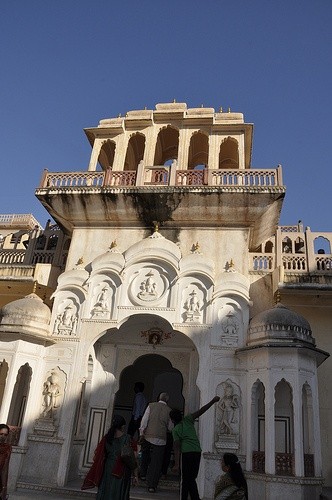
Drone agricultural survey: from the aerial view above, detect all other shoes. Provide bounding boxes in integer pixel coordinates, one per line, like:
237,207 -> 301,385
138,475 -> 146,480
147,486 -> 156,493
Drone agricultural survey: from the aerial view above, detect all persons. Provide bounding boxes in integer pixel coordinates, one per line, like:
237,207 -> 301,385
218,387 -> 238,434
81,415 -> 138,500
128,382 -> 147,441
139,393 -> 175,492
169,397 -> 220,500
44,375 -> 62,419
214,453 -> 248,500
0,424 -> 12,500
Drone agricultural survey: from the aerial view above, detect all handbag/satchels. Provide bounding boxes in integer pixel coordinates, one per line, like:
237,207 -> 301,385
111,455 -> 124,478
